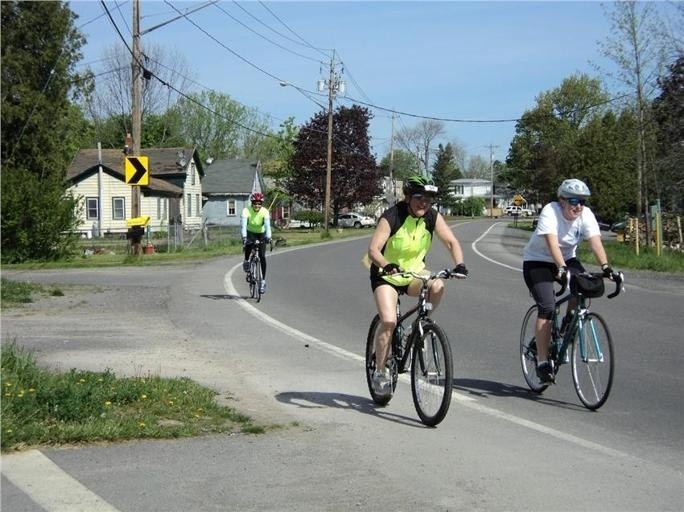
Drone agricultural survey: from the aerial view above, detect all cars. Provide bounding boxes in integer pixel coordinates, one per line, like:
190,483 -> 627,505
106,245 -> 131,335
611,211 -> 683,243
275,217 -> 316,229
330,212 -> 377,229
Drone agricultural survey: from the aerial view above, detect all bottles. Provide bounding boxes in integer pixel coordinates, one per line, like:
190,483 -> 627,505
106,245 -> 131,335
555,336 -> 570,364
400,324 -> 412,350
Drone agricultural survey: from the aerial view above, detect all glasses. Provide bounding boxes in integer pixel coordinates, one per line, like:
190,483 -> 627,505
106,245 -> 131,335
411,193 -> 434,204
254,202 -> 262,205
566,198 -> 586,206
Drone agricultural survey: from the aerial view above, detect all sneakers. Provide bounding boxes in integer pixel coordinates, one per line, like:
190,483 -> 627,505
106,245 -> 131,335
536,363 -> 557,383
404,322 -> 428,352
259,280 -> 266,293
559,318 -> 582,343
243,259 -> 250,271
371,368 -> 394,397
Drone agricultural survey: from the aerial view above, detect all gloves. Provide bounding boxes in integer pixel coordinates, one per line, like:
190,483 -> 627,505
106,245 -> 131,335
383,262 -> 403,273
556,265 -> 570,280
454,262 -> 468,275
602,263 -> 613,274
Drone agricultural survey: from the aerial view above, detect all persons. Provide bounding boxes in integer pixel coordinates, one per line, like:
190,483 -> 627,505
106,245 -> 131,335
239,192 -> 274,295
366,174 -> 468,398
521,178 -> 623,388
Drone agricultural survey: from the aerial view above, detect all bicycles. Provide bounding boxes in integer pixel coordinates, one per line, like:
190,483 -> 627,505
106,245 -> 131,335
520,269 -> 624,410
366,269 -> 467,426
243,240 -> 272,302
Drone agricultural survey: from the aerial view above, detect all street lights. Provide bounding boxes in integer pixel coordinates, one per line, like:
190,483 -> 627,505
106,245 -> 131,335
280,81 -> 333,233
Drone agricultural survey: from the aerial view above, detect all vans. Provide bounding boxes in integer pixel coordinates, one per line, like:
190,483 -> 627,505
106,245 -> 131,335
503,206 -> 523,216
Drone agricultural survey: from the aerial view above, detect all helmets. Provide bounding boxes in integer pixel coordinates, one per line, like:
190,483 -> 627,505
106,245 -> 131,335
250,192 -> 264,201
556,178 -> 591,201
402,174 -> 443,196
